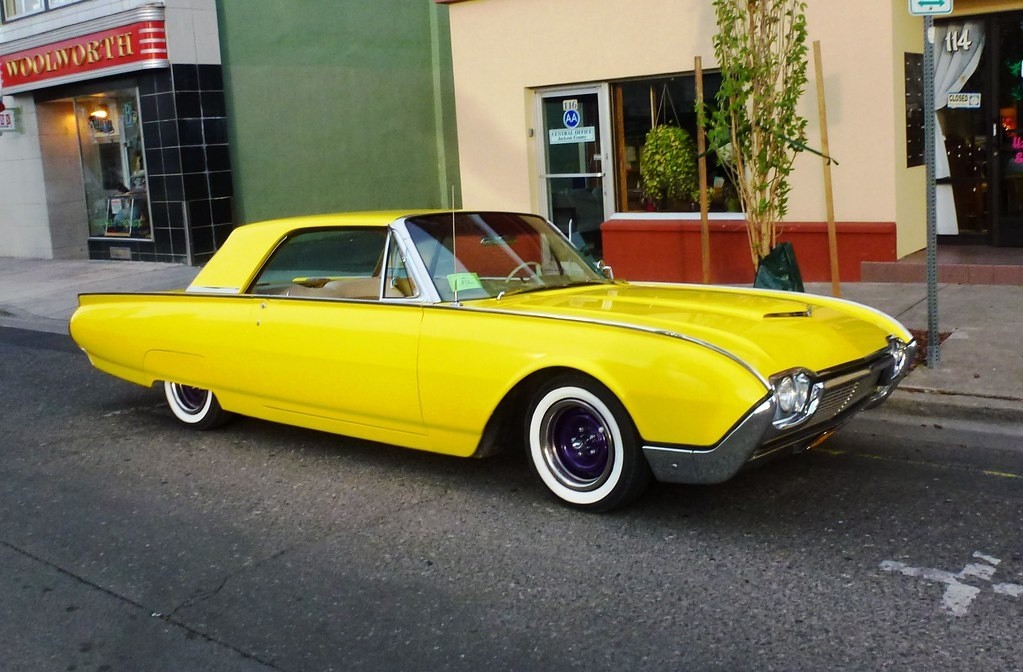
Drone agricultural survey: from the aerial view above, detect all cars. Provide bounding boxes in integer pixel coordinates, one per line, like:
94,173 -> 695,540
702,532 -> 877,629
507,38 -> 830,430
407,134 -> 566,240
70,209 -> 921,512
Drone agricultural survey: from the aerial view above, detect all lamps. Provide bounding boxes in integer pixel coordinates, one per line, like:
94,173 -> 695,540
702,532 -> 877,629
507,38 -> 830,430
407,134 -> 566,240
95,98 -> 107,118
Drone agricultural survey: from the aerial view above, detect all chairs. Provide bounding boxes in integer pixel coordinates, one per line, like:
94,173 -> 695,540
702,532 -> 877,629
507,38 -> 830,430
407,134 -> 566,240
263,277 -> 450,298
